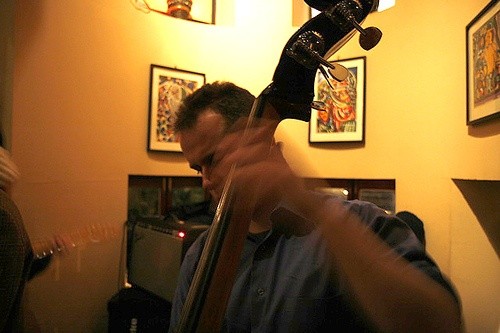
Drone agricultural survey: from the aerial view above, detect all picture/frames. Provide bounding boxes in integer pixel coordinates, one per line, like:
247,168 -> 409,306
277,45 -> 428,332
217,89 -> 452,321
308,56 -> 367,144
466,0 -> 500,126
146,64 -> 206,154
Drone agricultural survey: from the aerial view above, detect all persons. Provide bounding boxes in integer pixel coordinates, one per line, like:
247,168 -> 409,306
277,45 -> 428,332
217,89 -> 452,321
0,125 -> 35,333
169,80 -> 464,333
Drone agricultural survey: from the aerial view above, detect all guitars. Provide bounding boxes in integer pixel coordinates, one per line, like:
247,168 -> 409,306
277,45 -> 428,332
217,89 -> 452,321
27,236 -> 76,281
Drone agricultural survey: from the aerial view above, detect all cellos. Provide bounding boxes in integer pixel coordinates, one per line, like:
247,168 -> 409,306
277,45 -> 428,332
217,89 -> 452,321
174,0 -> 382,333
